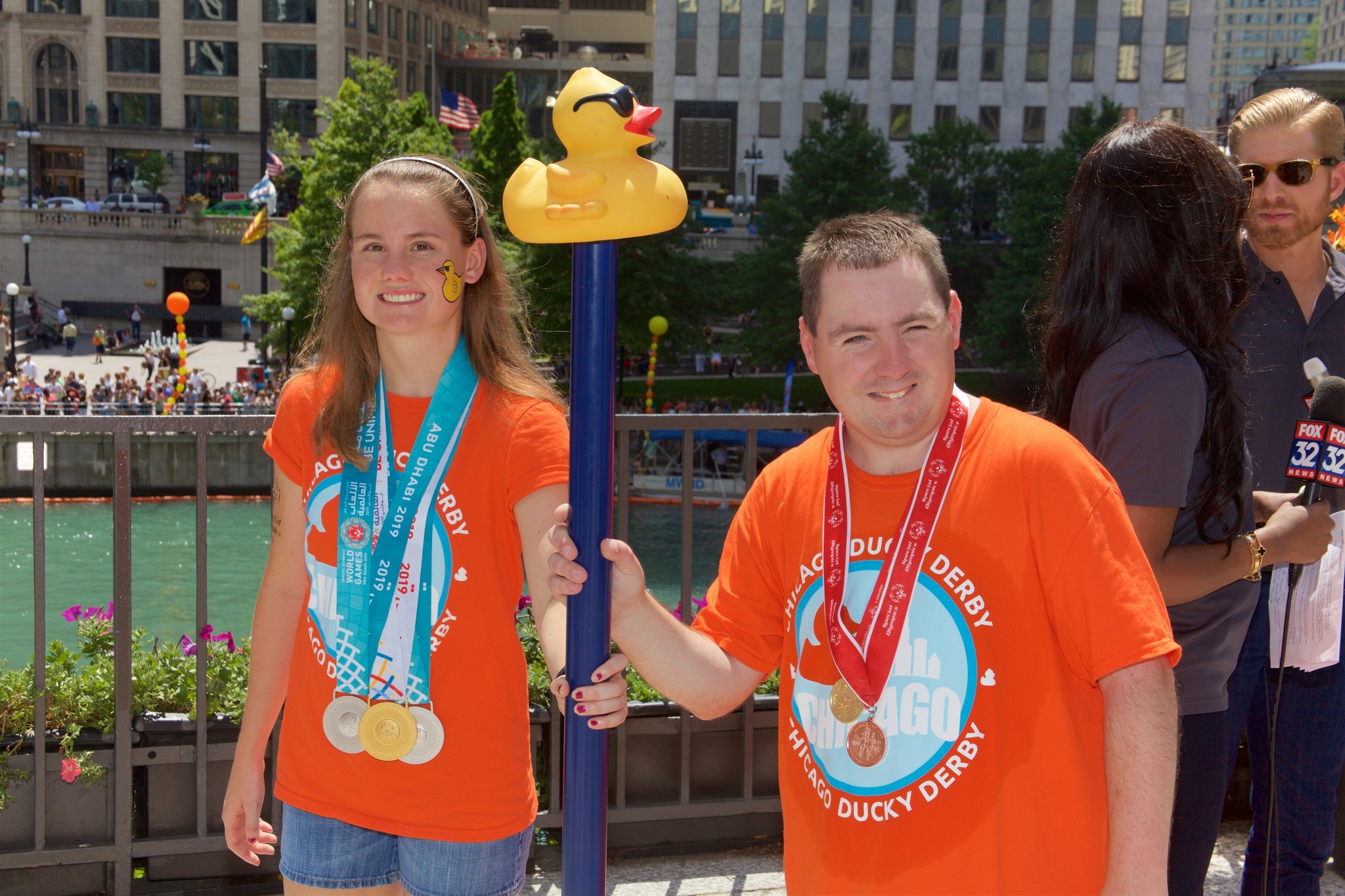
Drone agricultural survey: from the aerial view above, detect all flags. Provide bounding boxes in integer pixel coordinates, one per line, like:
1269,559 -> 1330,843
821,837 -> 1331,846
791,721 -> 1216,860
266,150 -> 285,176
240,206 -> 267,245
247,169 -> 271,204
438,88 -> 481,131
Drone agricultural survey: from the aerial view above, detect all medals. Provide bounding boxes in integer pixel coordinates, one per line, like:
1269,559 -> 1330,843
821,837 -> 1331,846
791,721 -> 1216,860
357,692 -> 419,762
397,700 -> 445,765
828,677 -> 863,724
322,689 -> 372,755
846,720 -> 887,767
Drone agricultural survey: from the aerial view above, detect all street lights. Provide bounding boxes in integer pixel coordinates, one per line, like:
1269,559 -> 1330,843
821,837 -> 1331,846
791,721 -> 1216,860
281,306 -> 296,381
5,282 -> 19,377
16,109 -> 40,208
20,234 -> 33,285
192,131 -> 212,195
742,143 -> 764,228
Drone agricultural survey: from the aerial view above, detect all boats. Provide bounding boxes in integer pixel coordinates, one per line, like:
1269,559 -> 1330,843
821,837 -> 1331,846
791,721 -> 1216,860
613,430 -> 810,508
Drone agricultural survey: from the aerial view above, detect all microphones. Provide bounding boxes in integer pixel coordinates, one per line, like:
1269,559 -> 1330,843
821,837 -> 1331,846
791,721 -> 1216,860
1286,376 -> 1345,590
1302,357 -> 1330,411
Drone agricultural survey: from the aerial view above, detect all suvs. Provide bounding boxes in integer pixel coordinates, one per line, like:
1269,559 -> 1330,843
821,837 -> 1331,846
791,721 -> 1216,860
97,193 -> 170,222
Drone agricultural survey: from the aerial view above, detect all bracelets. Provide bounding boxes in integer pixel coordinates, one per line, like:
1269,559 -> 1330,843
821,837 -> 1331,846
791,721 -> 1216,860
1237,530 -> 1266,582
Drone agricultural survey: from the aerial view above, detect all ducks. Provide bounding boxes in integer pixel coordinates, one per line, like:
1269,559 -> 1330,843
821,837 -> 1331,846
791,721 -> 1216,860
500,63 -> 691,245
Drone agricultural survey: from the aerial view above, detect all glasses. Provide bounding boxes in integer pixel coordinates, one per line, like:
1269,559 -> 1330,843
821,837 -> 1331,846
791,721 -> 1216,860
1233,156 -> 1341,189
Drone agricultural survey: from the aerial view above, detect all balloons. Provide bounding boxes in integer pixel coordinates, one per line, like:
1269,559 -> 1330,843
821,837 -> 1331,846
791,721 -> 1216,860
163,291 -> 190,415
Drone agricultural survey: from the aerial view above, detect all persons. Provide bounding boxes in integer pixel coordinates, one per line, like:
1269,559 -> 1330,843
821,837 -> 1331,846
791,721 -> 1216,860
0,293 -> 78,356
548,209 -> 1185,896
1031,116 -> 1335,896
95,189 -> 100,202
92,304 -> 146,364
219,157 -> 633,896
37,195 -> 48,209
127,205 -> 135,212
0,347 -> 282,416
158,193 -> 170,213
175,195 -> 186,214
53,200 -> 62,209
1210,85 -> 1345,896
111,201 -> 123,212
83,196 -> 100,212
240,311 -> 253,352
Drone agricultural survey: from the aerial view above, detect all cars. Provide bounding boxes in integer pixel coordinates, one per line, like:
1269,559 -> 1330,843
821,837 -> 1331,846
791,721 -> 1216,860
24,197 -> 86,220
189,192 -> 259,216
